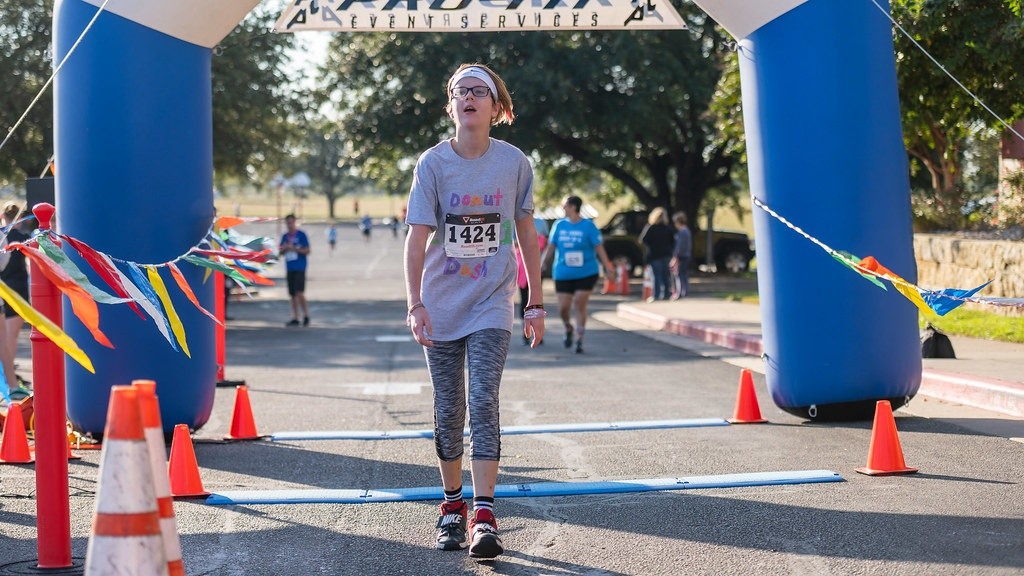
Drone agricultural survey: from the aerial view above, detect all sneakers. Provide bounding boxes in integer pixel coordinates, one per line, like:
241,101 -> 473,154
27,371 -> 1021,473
468,509 -> 503,558
435,500 -> 469,551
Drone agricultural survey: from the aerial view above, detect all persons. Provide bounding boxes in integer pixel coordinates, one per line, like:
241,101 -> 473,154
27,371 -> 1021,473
326,223 -> 337,250
515,233 -> 546,346
0,200 -> 32,399
391,215 -> 399,237
403,64 -> 545,559
362,215 -> 372,240
670,212 -> 691,301
279,215 -> 311,327
639,206 -> 675,303
541,196 -> 612,353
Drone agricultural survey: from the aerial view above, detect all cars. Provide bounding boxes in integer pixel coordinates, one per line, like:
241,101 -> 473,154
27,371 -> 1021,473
599,210 -> 756,276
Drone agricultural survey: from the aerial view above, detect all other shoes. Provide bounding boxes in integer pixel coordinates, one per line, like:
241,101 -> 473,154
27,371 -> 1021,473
564,326 -> 573,348
285,319 -> 299,326
303,315 -> 309,327
575,338 -> 584,354
539,338 -> 543,345
523,332 -> 530,346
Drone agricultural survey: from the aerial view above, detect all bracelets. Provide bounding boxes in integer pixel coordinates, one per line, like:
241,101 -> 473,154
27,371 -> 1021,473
409,302 -> 424,314
524,304 -> 545,319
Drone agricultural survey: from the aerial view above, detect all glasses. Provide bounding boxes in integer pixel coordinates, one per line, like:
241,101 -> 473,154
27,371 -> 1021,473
451,86 -> 496,104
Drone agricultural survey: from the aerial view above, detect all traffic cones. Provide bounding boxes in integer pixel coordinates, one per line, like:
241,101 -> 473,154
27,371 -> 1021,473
614,266 -> 631,296
223,385 -> 274,441
131,379 -> 186,576
642,263 -> 654,301
83,386 -> 170,576
67,436 -> 80,460
0,402 -> 34,465
725,367 -> 769,424
853,399 -> 921,477
600,272 -> 616,295
165,421 -> 211,499
